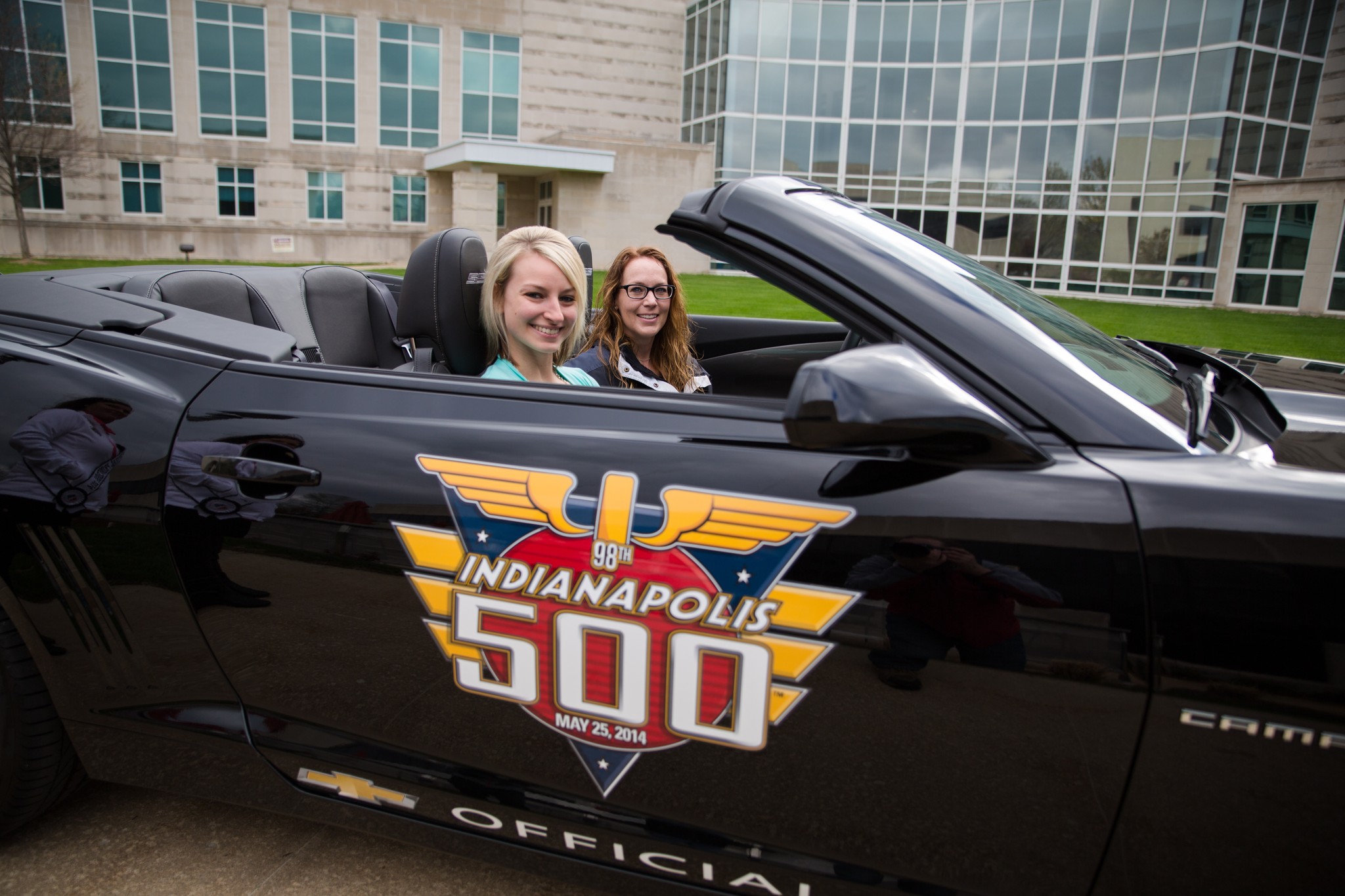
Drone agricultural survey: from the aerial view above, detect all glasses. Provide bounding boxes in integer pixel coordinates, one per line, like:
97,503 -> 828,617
617,284 -> 675,300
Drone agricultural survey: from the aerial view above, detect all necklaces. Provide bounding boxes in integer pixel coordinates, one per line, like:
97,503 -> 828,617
636,357 -> 651,362
553,368 -> 568,385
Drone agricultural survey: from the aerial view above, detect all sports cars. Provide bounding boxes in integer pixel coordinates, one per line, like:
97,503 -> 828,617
0,176 -> 1345,896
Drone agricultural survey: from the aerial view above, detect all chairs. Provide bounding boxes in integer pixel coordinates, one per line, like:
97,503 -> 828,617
397,233 -> 499,384
125,265 -> 304,358
295,261 -> 424,378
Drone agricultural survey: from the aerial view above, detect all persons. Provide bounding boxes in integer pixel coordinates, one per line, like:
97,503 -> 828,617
475,225 -> 601,385
567,243 -> 714,397
